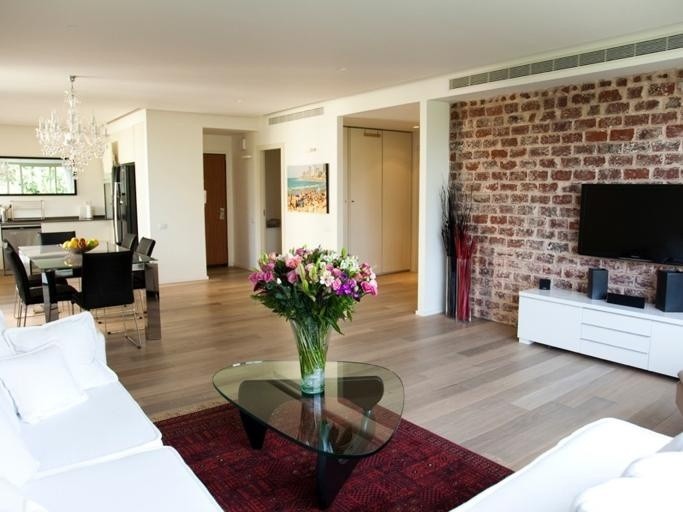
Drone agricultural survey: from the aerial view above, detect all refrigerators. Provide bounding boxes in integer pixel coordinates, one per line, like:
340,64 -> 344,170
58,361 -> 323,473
111,163 -> 137,249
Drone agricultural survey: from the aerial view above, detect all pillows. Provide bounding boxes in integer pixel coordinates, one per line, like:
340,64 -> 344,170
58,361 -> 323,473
0,337 -> 88,424
3,309 -> 120,389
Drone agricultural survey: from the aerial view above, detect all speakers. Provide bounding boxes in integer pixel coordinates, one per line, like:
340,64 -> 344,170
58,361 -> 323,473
588,269 -> 608,299
655,270 -> 683,312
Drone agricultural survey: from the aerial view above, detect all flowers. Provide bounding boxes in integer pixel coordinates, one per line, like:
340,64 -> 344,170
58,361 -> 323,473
239,242 -> 381,384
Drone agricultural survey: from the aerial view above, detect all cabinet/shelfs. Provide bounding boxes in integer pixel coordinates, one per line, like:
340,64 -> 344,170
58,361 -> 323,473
513,284 -> 682,380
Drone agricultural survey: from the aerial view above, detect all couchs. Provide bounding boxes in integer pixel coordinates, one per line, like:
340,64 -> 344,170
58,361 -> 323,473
447,408 -> 682,511
0,306 -> 229,511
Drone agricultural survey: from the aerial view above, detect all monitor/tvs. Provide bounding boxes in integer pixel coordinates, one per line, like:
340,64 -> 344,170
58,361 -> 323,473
577,183 -> 683,265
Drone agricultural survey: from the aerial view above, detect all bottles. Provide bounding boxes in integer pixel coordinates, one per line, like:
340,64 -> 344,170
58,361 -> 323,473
77,201 -> 96,220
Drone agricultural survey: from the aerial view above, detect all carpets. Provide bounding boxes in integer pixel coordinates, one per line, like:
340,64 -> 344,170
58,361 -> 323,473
151,398 -> 515,511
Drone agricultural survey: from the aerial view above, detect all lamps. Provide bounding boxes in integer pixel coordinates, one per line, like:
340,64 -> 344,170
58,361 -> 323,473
30,73 -> 116,183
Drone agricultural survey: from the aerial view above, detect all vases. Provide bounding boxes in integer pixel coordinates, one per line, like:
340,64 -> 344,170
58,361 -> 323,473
443,253 -> 455,320
454,256 -> 473,326
291,313 -> 331,397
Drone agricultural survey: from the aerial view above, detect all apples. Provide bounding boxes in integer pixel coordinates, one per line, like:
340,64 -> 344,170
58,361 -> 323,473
64,238 -> 98,248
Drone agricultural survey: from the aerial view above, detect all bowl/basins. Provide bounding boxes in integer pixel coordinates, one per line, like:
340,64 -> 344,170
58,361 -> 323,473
63,254 -> 82,266
55,244 -> 99,253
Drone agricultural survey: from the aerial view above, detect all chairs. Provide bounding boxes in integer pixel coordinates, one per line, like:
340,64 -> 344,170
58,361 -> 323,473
0,228 -> 163,351
